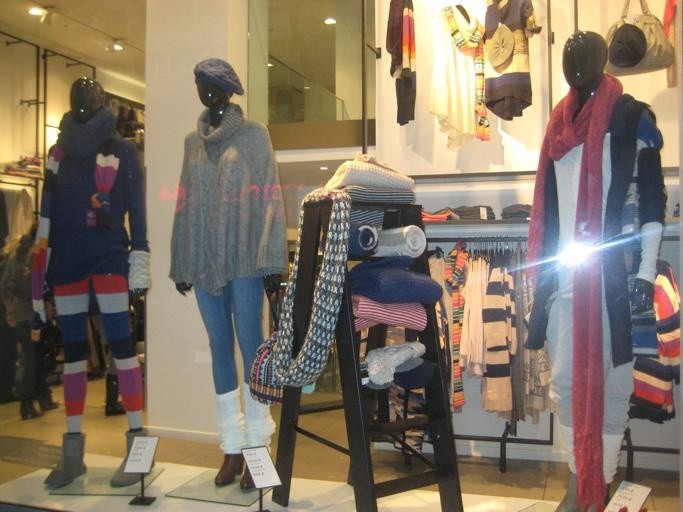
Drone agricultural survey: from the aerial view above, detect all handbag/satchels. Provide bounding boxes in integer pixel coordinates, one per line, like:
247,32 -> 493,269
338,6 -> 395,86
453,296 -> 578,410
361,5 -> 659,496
605,14 -> 675,77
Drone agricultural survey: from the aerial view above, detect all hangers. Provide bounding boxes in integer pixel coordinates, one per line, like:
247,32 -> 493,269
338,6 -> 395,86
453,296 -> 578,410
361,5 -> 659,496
467,232 -> 527,263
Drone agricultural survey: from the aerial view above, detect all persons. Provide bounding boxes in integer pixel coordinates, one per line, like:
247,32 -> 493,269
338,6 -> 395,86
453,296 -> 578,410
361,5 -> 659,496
517,31 -> 667,511
165,57 -> 289,493
33,76 -> 156,489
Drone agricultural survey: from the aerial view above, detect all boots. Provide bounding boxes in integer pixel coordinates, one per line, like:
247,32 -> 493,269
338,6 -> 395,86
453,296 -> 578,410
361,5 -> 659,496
105,373 -> 125,416
44,433 -> 87,485
111,428 -> 155,487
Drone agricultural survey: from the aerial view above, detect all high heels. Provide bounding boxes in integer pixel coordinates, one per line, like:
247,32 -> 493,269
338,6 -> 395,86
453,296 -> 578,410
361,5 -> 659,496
20,399 -> 44,420
215,453 -> 244,486
38,395 -> 59,411
240,463 -> 255,489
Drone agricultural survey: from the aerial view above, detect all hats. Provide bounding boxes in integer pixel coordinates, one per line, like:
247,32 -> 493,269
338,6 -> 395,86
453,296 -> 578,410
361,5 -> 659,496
364,341 -> 426,387
193,58 -> 244,95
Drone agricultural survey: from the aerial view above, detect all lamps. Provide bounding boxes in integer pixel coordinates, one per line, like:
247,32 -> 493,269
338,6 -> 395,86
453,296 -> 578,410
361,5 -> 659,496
105,40 -> 125,53
29,7 -> 54,24
